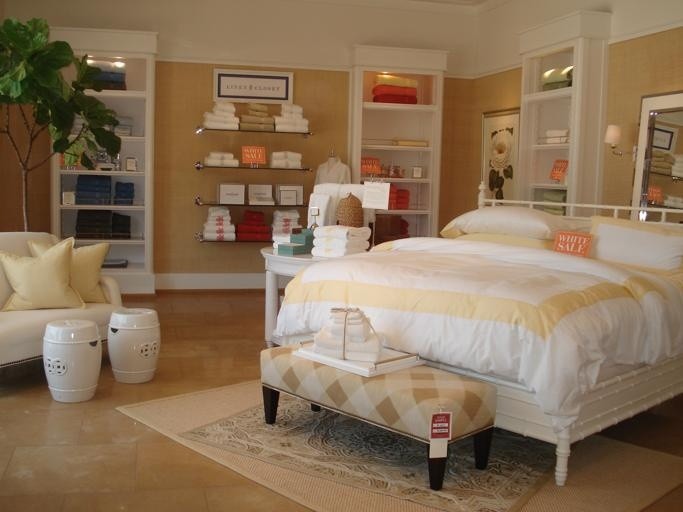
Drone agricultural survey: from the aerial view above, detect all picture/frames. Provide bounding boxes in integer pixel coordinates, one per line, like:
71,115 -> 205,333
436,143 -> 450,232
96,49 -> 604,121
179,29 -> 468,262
481,106 -> 520,206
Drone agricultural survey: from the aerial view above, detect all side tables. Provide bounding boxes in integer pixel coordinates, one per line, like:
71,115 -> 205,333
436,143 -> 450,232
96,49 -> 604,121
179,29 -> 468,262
260,246 -> 366,348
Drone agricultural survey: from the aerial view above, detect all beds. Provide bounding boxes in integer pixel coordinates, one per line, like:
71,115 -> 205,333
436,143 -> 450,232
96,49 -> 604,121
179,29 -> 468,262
270,181 -> 683,486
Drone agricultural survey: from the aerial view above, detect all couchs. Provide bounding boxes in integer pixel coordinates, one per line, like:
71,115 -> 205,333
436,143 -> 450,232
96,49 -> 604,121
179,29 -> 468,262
1,232 -> 124,367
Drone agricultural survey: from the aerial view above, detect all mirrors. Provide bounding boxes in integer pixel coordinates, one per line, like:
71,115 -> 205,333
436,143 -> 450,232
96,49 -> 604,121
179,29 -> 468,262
630,90 -> 683,224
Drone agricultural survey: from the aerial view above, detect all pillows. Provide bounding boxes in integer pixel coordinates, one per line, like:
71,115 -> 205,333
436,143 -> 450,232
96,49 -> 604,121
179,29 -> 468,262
0,237 -> 110,312
440,206 -> 573,241
453,233 -> 557,250
586,216 -> 683,274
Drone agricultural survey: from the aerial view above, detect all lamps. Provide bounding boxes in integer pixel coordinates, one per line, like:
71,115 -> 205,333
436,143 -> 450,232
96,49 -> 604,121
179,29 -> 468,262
604,124 -> 638,159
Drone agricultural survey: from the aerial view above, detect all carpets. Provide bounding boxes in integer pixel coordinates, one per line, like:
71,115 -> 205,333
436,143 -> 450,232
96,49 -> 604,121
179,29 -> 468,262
115,379 -> 539,512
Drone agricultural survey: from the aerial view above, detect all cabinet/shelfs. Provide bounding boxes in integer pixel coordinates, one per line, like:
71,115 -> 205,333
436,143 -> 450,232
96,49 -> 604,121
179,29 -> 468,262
48,26 -> 159,295
517,11 -> 613,223
349,46 -> 448,252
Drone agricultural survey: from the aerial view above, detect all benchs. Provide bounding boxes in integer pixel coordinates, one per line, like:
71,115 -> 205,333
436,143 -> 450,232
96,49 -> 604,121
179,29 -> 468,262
259,342 -> 498,491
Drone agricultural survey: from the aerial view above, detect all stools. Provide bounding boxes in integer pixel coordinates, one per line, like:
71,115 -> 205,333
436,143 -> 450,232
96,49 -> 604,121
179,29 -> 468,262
107,308 -> 161,384
42,319 -> 102,403
